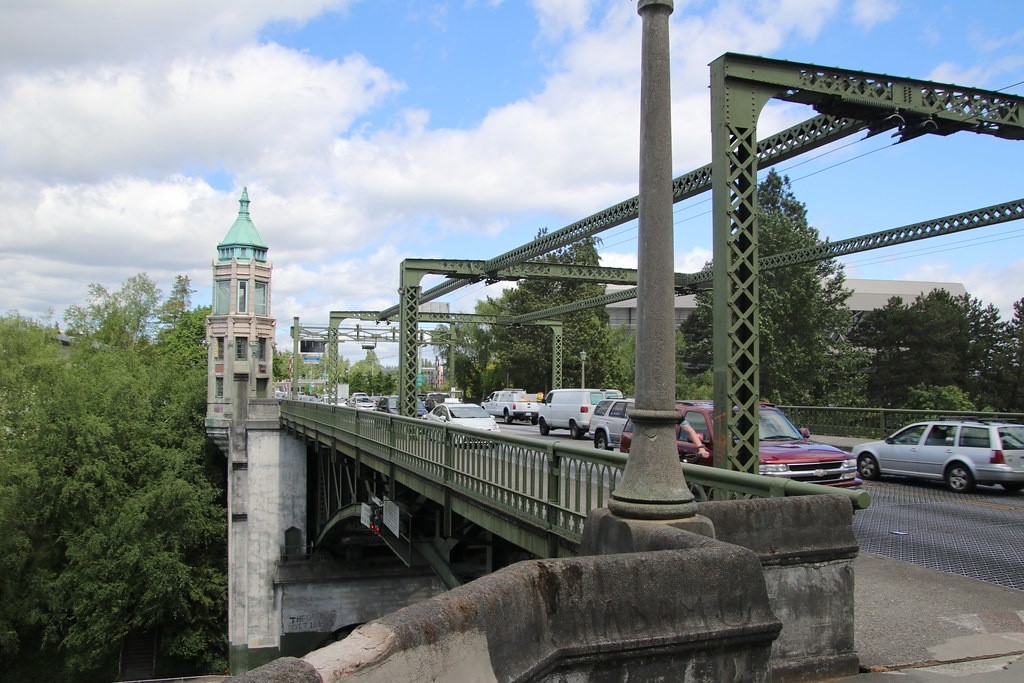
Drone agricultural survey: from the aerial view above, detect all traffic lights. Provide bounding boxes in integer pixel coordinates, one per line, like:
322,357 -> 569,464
370,506 -> 383,534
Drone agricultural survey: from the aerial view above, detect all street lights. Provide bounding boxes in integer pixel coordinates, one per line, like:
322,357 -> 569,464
579,349 -> 587,389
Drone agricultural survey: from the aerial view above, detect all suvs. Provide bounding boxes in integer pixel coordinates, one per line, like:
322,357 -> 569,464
588,396 -> 635,450
850,415 -> 1024,492
619,400 -> 863,488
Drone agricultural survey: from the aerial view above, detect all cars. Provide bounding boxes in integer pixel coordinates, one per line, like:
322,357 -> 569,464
275,391 -> 342,403
347,392 -> 369,407
375,397 -> 428,434
422,393 -> 464,411
427,403 -> 501,447
352,396 -> 378,419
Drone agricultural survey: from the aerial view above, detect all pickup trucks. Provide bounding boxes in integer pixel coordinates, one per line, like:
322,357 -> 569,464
481,391 -> 543,425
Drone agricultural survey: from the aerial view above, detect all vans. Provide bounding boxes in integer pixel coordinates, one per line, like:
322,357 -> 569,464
538,389 -> 623,439
